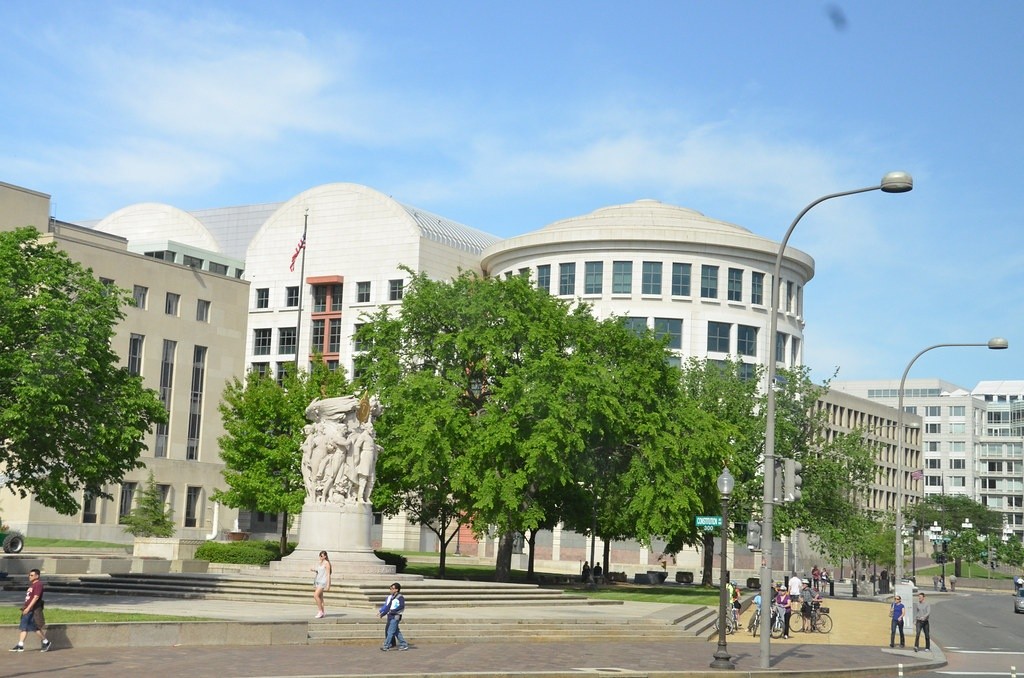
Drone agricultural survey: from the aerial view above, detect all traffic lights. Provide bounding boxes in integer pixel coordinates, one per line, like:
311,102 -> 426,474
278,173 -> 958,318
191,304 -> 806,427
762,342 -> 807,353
992,548 -> 997,560
936,553 -> 947,564
785,459 -> 803,502
942,542 -> 948,551
991,561 -> 995,569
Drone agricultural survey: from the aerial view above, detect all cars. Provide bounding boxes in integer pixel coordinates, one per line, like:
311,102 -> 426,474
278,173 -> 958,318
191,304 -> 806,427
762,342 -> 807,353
1012,588 -> 1024,614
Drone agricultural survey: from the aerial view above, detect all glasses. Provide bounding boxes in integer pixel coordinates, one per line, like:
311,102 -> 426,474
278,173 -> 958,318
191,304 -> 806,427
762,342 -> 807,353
895,598 -> 899,600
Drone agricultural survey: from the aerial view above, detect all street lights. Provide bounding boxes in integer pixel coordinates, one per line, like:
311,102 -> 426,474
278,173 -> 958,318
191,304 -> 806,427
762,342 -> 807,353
709,467 -> 736,671
758,171 -> 913,670
894,337 -> 1008,587
851,423 -> 920,597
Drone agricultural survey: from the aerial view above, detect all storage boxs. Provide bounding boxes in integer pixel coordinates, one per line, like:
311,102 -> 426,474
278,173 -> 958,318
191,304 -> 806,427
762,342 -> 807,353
819,607 -> 830,613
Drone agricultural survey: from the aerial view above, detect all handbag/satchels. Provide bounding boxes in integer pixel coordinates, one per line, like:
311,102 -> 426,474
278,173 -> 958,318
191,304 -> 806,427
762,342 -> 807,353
889,604 -> 895,617
34,607 -> 46,627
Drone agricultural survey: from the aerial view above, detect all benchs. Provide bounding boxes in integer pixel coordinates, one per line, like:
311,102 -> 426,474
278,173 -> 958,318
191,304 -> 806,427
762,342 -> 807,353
535,574 -> 604,585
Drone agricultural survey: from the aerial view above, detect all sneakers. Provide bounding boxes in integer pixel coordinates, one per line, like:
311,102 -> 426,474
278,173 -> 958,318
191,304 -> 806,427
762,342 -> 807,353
8,645 -> 24,652
41,641 -> 52,652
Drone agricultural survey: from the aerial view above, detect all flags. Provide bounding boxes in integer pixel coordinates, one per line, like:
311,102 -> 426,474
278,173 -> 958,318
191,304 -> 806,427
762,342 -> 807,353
289,232 -> 305,272
911,468 -> 924,480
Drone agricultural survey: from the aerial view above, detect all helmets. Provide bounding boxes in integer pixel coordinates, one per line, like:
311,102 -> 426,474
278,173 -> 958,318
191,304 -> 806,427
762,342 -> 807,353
802,579 -> 809,584
730,580 -> 737,586
780,586 -> 786,590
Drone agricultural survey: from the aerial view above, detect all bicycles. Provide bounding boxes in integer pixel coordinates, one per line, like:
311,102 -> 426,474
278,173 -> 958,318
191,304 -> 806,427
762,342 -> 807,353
749,598 -> 833,639
714,598 -> 739,635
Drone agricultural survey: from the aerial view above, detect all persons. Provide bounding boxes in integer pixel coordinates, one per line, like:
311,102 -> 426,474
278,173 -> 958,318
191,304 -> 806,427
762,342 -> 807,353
376,582 -> 408,651
745,578 -> 791,638
890,595 -> 905,647
1014,574 -> 1024,591
310,551 -> 332,618
949,573 -> 957,591
890,572 -> 895,587
901,575 -> 906,579
812,564 -> 821,591
699,571 -> 703,580
913,592 -> 931,652
8,568 -> 51,652
593,562 -> 602,583
299,413 -> 384,504
819,568 -> 829,593
799,578 -> 819,633
932,574 -> 939,591
939,575 -> 943,583
811,586 -> 823,630
583,561 -> 590,572
788,572 -> 803,613
880,567 -> 888,579
713,577 -> 742,634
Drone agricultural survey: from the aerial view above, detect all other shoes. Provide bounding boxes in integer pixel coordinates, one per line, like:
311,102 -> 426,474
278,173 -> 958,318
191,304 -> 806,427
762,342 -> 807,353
801,629 -> 817,634
925,649 -> 929,652
914,647 -> 918,651
380,644 -> 409,651
890,644 -> 894,647
784,635 -> 788,638
899,645 -> 903,647
